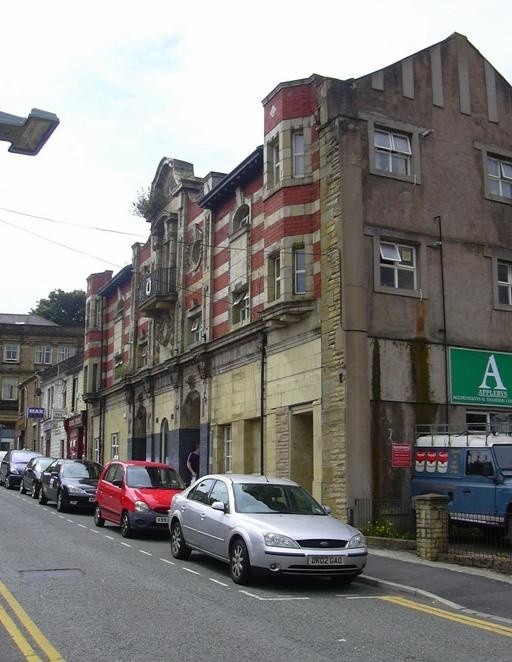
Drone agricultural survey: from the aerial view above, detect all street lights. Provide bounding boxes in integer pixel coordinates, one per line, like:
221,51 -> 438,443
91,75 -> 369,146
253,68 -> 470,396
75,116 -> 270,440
0,108 -> 58,155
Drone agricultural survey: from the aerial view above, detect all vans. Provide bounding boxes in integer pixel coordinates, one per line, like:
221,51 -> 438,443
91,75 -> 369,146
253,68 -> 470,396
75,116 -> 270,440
94,459 -> 186,538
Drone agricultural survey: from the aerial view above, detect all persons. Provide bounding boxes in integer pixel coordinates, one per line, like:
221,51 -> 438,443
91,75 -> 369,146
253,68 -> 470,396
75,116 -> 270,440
187,443 -> 200,484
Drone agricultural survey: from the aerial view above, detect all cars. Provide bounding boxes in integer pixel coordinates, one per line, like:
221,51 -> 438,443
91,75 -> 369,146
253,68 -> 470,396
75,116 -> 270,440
165,474 -> 368,590
0,448 -> 104,516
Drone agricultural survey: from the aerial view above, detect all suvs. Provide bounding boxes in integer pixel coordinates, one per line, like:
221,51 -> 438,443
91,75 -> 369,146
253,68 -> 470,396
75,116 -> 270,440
408,419 -> 512,546
